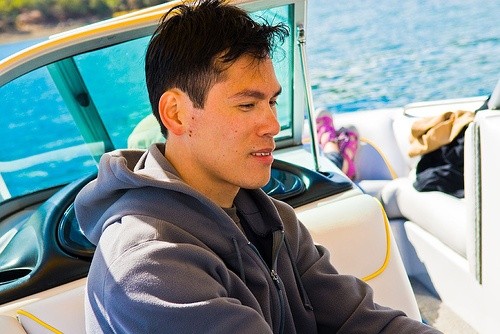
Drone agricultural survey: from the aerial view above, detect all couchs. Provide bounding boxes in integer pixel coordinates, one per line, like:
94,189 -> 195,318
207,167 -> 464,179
313,94 -> 500,334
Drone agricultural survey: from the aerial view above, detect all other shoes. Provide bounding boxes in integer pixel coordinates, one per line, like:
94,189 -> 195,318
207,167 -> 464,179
317,110 -> 337,153
338,128 -> 358,181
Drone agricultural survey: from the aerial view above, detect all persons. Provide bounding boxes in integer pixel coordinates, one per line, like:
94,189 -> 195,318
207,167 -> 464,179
72,2 -> 447,334
126,106 -> 361,184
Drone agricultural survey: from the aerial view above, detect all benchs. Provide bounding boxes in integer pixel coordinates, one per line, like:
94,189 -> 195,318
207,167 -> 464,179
0,187 -> 426,334
